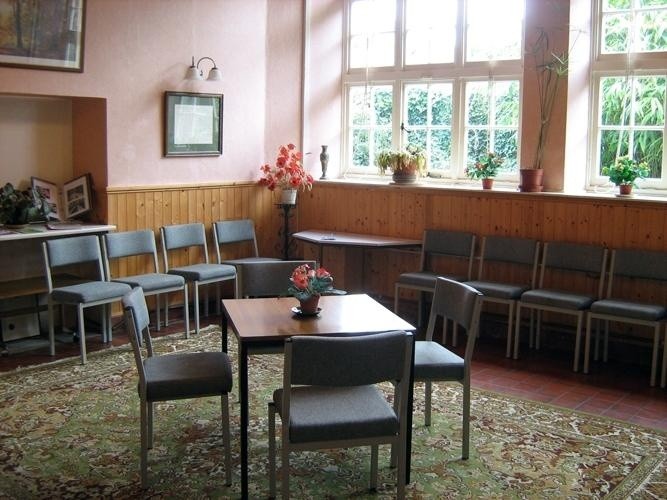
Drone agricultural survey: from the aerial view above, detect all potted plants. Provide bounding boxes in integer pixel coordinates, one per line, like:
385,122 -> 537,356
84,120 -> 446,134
515,25 -> 588,193
372,142 -> 431,185
2,179 -> 52,230
289,265 -> 334,316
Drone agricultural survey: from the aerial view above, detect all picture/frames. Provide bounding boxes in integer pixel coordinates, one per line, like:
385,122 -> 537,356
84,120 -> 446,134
162,91 -> 225,159
0,1 -> 89,75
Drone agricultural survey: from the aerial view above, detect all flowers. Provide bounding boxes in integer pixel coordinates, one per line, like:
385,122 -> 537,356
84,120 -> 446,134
255,140 -> 314,193
601,154 -> 651,189
462,147 -> 509,179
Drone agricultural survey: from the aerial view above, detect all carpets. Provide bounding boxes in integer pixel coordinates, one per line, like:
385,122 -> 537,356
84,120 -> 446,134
27,324 -> 667,500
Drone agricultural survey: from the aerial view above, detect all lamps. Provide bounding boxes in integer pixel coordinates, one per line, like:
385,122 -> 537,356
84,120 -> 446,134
184,55 -> 224,85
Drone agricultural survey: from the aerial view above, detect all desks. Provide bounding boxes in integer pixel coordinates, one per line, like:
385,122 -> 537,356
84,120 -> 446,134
219,293 -> 415,500
294,228 -> 424,295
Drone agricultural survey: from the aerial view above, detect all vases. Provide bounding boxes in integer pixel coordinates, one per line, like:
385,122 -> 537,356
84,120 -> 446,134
618,183 -> 632,196
270,189 -> 297,205
480,178 -> 494,189
318,145 -> 329,180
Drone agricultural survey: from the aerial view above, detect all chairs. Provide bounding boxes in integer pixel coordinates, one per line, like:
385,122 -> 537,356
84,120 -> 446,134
41,233 -> 140,365
452,236 -> 542,361
121,286 -> 234,492
100,229 -> 191,346
515,241 -> 609,373
415,278 -> 484,460
584,247 -> 667,388
267,330 -> 414,500
159,222 -> 238,334
212,220 -> 283,321
395,230 -> 476,346
237,260 -> 317,424
